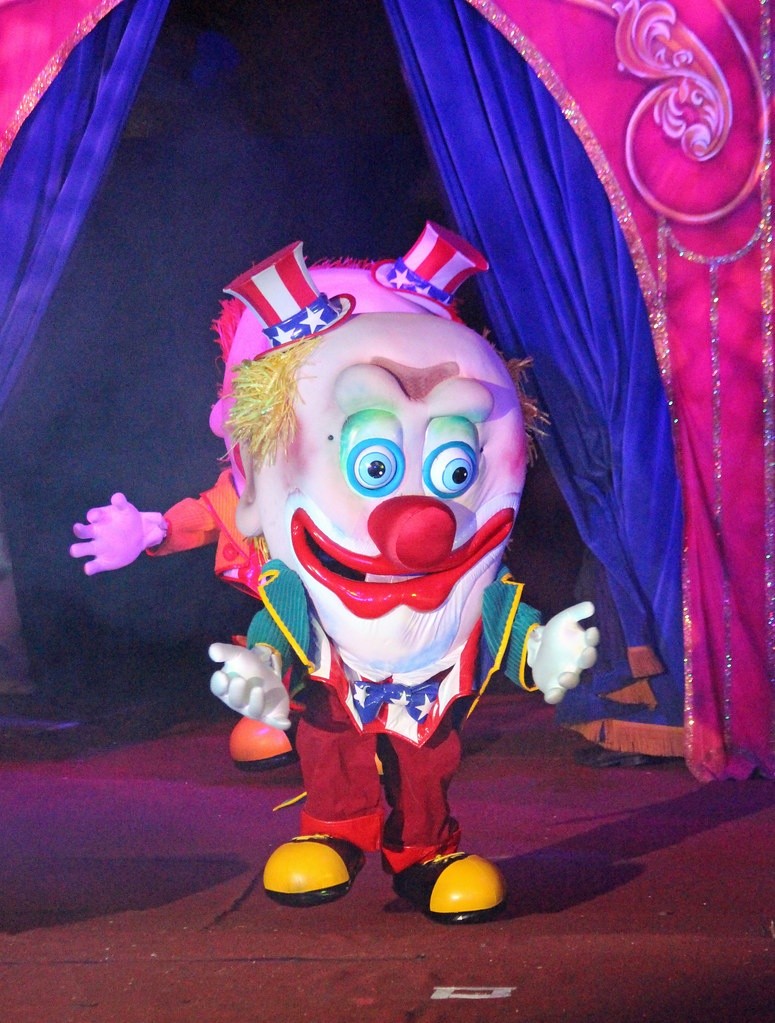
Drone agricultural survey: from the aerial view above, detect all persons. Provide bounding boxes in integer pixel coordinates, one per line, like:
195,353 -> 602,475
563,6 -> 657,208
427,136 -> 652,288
70,220 -> 491,772
208,241 -> 601,923
